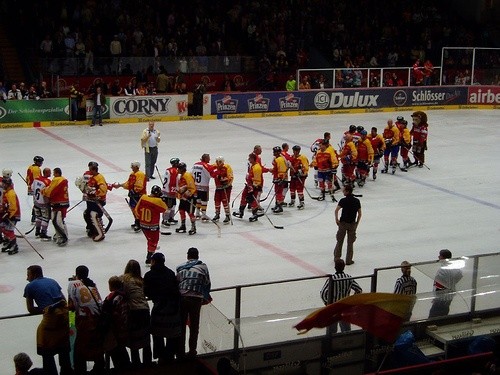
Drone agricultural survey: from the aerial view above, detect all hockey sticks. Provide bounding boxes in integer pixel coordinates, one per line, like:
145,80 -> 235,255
92,198 -> 113,235
287,163 -> 322,199
3,213 -> 45,260
174,190 -> 223,238
154,165 -> 164,189
334,173 -> 363,197
17,172 -> 28,185
385,147 -> 409,172
256,183 -> 284,217
231,186 -> 245,208
244,183 -> 286,229
256,181 -> 279,203
125,197 -> 160,250
127,194 -> 173,236
399,143 -> 430,171
220,183 -> 233,225
24,225 -> 37,235
65,199 -> 85,214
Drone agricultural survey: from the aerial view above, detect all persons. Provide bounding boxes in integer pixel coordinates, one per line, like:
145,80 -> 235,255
0,156 -> 70,255
162,110 -> 416,235
429,249 -> 463,318
13,248 -> 235,375
79,161 -> 146,242
141,120 -> 160,182
334,185 -> 362,265
135,185 -> 168,267
0,0 -> 500,127
320,259 -> 363,334
394,261 -> 418,296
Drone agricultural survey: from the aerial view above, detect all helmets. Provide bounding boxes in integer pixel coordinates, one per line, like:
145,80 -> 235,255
88,115 -> 406,196
33,156 -> 43,163
1,167 -> 12,184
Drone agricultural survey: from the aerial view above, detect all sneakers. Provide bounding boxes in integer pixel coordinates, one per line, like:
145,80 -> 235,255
233,212 -> 244,218
1,244 -> 11,252
168,217 -> 178,224
60,237 -> 69,247
39,233 -> 52,241
189,224 -> 196,235
289,200 -> 295,207
131,223 -> 141,232
223,215 -> 230,225
34,232 -> 40,237
161,219 -> 170,227
318,160 -> 423,202
248,214 -> 258,222
271,204 -> 283,215
176,224 -> 186,233
281,202 -> 287,207
213,213 -> 220,222
8,244 -> 18,255
297,201 -> 304,210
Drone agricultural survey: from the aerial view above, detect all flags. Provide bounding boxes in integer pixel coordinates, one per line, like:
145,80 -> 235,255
292,292 -> 417,345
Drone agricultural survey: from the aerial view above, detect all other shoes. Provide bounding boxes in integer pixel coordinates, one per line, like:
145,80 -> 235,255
196,214 -> 210,223
99,123 -> 103,126
90,123 -> 94,126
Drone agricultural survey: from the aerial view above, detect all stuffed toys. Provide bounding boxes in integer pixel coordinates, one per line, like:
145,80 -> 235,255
409,111 -> 428,167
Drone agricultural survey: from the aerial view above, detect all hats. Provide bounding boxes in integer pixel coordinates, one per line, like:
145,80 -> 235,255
150,252 -> 166,262
